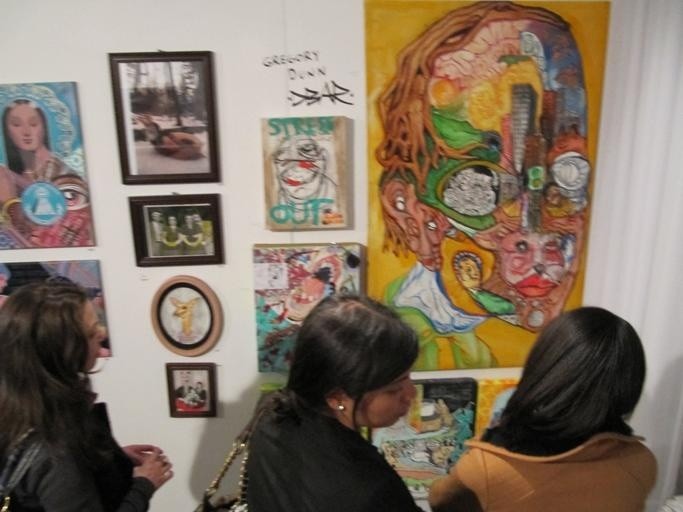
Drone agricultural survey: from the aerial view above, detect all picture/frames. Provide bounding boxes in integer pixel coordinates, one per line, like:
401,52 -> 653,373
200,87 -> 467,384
165,362 -> 219,419
126,193 -> 224,268
150,275 -> 224,357
108,53 -> 222,186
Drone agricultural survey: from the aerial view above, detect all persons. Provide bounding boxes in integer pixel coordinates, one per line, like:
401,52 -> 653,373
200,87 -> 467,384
427,306 -> 659,511
241,290 -> 426,511
1,274 -> 174,512
1,99 -> 95,251
